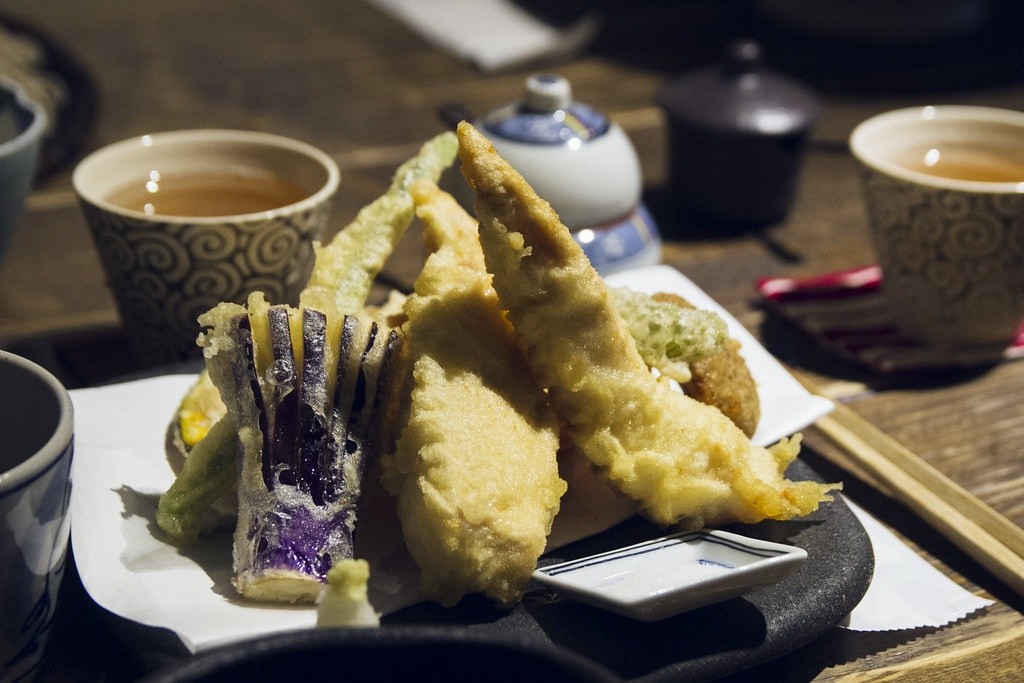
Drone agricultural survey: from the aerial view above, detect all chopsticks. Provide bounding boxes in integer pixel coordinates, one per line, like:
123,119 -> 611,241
782,370 -> 1024,596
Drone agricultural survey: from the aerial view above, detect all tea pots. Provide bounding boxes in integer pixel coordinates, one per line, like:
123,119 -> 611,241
436,71 -> 665,283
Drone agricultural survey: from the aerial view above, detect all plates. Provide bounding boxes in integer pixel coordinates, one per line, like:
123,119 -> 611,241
66,264 -> 872,682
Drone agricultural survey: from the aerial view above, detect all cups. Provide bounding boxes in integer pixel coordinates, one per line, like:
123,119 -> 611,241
0,74 -> 48,246
656,43 -> 817,237
847,102 -> 1024,347
0,349 -> 76,683
71,128 -> 341,362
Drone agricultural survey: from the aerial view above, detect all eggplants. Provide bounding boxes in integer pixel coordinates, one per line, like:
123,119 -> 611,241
201,304 -> 403,602
